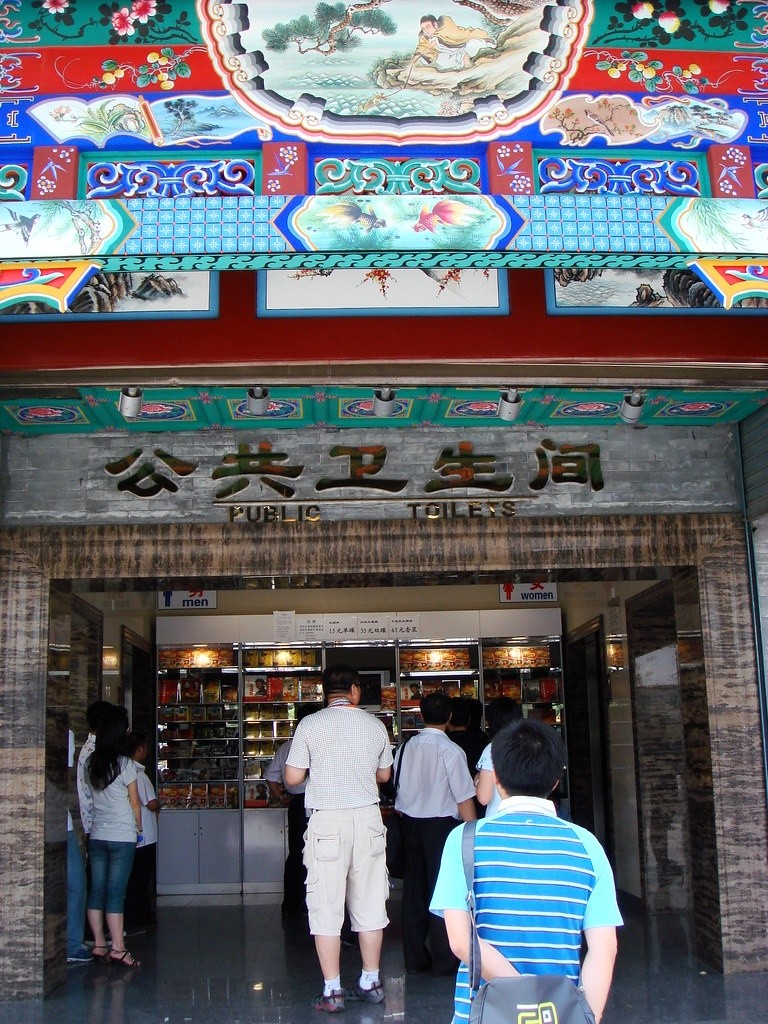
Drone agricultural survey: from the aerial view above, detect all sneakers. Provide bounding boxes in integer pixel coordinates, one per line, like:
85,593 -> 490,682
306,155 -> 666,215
312,989 -> 346,1013
84,931 -> 116,944
66,949 -> 95,962
343,975 -> 386,1003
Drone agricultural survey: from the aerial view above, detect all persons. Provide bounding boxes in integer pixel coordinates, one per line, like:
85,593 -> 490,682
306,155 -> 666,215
392,693 -> 476,976
255,679 -> 267,695
285,663 -> 392,1013
65,702 -> 161,969
410,684 -> 421,699
429,720 -> 624,1023
474,697 -> 522,817
256,784 -> 267,800
84,965 -> 135,1024
263,704 -> 319,915
45,712 -> 66,919
447,698 -> 488,779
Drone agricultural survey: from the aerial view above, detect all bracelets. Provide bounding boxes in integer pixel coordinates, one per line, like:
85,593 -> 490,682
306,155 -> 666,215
139,830 -> 143,831
475,779 -> 479,786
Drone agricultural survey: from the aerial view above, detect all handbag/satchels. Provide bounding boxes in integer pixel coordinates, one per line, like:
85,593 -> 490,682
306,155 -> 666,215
468,973 -> 597,1024
384,812 -> 404,879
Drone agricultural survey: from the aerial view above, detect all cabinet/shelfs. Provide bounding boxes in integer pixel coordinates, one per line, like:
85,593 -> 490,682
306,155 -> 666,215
154,634 -> 573,894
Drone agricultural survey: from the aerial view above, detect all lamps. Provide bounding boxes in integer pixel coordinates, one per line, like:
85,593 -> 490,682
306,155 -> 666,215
620,388 -> 645,424
373,387 -> 397,418
497,387 -> 522,422
247,387 -> 271,415
118,386 -> 143,418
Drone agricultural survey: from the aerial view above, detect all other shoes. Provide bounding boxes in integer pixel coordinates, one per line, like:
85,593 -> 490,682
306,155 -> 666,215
406,967 -> 428,974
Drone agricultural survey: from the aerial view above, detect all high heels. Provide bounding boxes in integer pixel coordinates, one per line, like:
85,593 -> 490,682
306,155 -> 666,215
110,949 -> 142,968
92,946 -> 113,963
